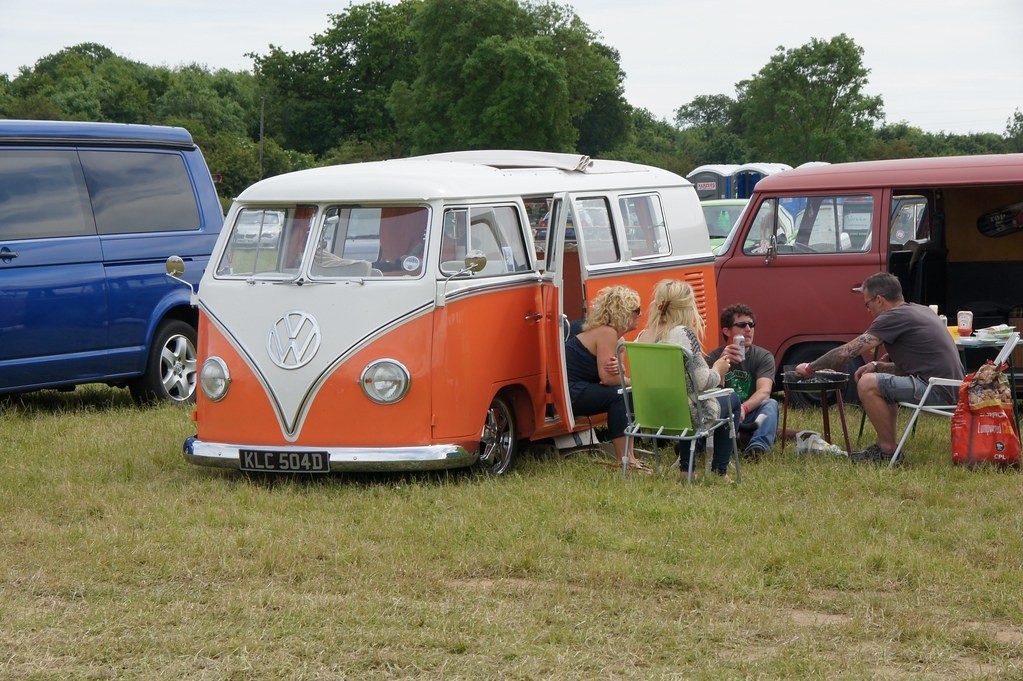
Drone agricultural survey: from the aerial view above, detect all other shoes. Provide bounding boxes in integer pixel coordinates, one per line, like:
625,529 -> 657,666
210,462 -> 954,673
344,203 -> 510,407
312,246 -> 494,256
851,444 -> 909,468
617,460 -> 653,475
743,446 -> 764,464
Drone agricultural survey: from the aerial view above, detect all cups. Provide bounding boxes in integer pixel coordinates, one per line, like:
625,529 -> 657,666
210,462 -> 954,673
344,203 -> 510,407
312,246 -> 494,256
929,305 -> 938,315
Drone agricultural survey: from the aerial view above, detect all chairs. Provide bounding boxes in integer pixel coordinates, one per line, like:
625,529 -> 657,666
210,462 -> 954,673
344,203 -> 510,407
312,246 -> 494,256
890,243 -> 906,254
134,175 -> 179,230
887,331 -> 1021,469
616,342 -> 743,487
904,238 -> 929,268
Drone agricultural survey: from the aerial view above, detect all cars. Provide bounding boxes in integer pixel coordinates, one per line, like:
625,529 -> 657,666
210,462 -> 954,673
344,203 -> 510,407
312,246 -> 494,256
313,196 -> 646,263
700,199 -> 798,261
861,195 -> 930,251
231,209 -> 285,250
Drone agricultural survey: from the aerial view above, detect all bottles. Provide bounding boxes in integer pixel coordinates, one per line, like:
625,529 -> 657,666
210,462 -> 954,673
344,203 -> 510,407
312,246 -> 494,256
957,311 -> 973,337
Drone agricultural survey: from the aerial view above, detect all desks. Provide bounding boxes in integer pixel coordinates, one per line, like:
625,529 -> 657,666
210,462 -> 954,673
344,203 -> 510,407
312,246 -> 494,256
856,335 -> 1023,442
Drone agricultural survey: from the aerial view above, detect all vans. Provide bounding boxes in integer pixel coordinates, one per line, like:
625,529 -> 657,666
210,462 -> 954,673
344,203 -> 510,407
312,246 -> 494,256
714,152 -> 1023,411
0,119 -> 234,410
165,149 -> 721,485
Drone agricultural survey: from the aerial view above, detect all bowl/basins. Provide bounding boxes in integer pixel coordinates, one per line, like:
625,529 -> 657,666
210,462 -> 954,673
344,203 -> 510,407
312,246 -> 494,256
973,326 -> 1021,344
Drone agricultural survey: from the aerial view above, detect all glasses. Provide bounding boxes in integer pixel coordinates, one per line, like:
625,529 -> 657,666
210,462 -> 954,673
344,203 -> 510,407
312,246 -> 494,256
633,307 -> 640,315
864,294 -> 885,308
730,322 -> 755,328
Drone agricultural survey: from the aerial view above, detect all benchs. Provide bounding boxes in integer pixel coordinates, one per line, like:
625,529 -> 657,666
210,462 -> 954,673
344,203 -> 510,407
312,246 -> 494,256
536,251 -> 584,322
911,247 -> 977,370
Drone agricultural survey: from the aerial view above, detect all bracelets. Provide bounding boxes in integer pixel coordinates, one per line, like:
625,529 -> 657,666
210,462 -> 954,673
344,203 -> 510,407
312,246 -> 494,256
741,404 -> 749,413
805,363 -> 815,375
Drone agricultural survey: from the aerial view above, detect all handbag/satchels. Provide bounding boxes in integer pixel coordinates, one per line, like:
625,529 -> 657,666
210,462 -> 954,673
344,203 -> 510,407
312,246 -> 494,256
796,429 -> 848,457
951,360 -> 1021,468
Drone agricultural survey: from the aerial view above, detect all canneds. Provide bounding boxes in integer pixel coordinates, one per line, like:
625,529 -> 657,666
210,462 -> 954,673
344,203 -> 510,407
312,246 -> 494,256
938,314 -> 947,327
989,324 -> 1009,334
732,335 -> 745,361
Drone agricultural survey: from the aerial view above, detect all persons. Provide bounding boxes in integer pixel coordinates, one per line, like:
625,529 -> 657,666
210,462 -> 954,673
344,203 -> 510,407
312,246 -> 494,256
795,273 -> 965,462
545,285 -> 653,476
634,279 -> 741,483
704,303 -> 778,463
